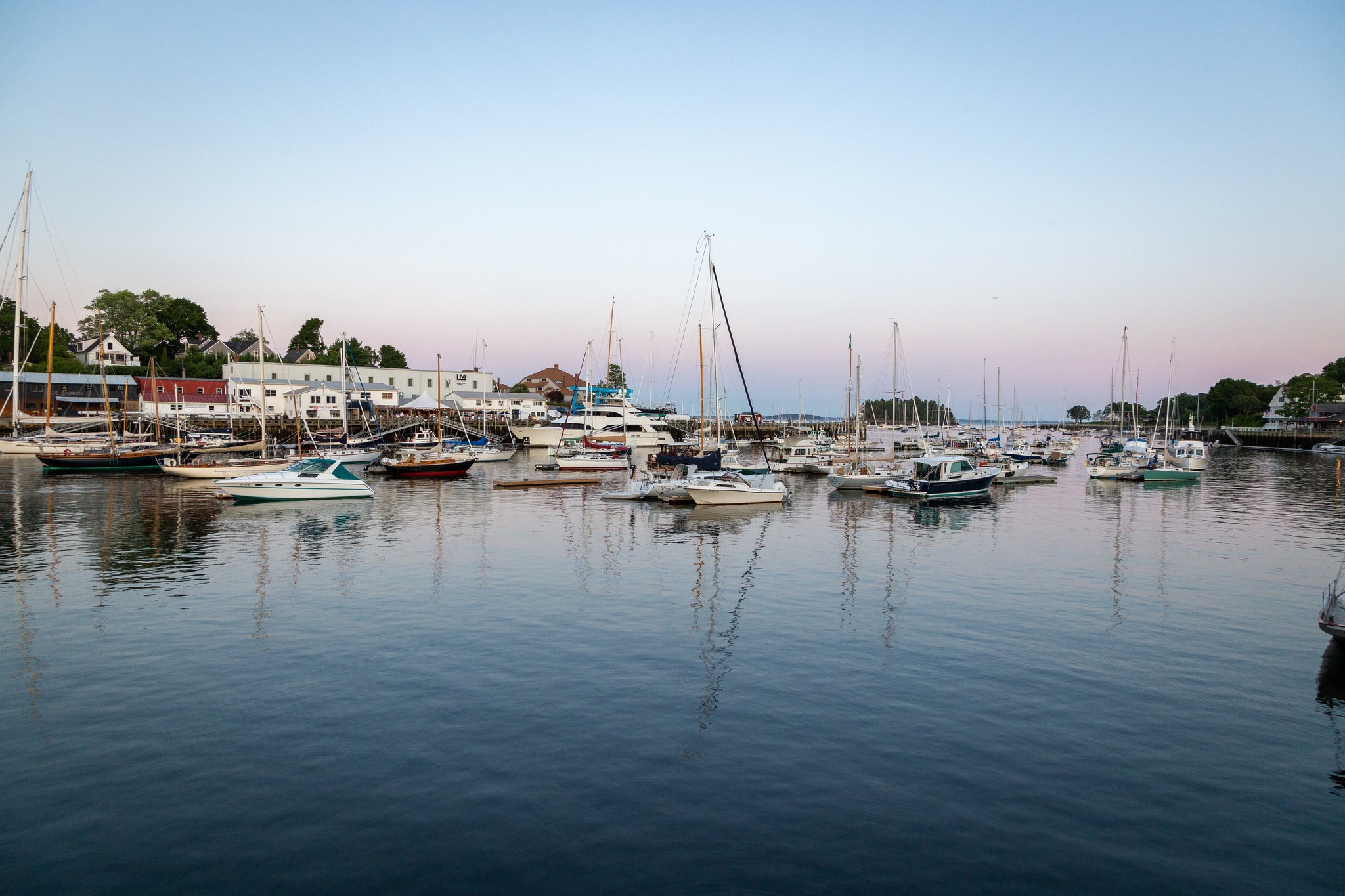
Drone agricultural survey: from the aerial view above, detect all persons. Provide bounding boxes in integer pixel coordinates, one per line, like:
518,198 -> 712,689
528,414 -> 531,422
748,437 -> 750,441
531,415 -> 534,420
414,412 -> 437,420
384,412 -> 392,418
487,415 -> 511,422
396,411 -> 411,418
824,463 -> 826,466
534,415 -> 537,420
516,418 -> 519,420
461,413 -> 481,420
994,452 -> 998,460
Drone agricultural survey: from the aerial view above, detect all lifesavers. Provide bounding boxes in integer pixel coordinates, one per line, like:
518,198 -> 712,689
825,435 -> 826,437
64,449 -> 72,455
167,459 -> 176,467
954,443 -> 958,448
837,468 -> 844,475
838,440 -> 841,443
1078,439 -> 1080,441
786,457 -> 789,461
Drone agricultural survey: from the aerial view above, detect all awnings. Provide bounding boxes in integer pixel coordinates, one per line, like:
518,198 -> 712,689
349,401 -> 371,409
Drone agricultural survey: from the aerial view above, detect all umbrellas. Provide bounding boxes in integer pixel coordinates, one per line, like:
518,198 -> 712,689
475,409 -> 487,417
486,410 -> 497,417
499,411 -> 510,414
398,390 -> 453,409
511,412 -> 522,419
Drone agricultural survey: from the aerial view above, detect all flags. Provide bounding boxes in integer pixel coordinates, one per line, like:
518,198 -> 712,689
394,433 -> 397,441
860,402 -> 864,411
1015,421 -> 1020,427
1264,410 -> 1271,417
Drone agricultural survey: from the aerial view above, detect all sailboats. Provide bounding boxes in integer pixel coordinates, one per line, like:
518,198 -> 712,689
0,164 -> 1215,502
